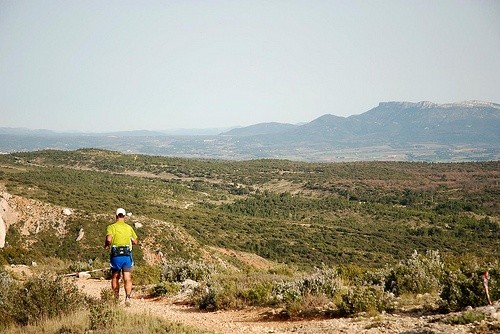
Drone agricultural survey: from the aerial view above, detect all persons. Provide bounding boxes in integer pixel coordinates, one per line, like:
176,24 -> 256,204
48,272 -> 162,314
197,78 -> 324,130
104,207 -> 138,308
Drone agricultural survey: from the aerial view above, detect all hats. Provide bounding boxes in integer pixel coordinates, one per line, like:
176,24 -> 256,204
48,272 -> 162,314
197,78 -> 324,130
116,208 -> 127,216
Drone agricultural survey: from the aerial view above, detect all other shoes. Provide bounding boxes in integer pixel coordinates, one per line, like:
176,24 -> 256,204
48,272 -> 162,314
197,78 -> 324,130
125,297 -> 131,306
